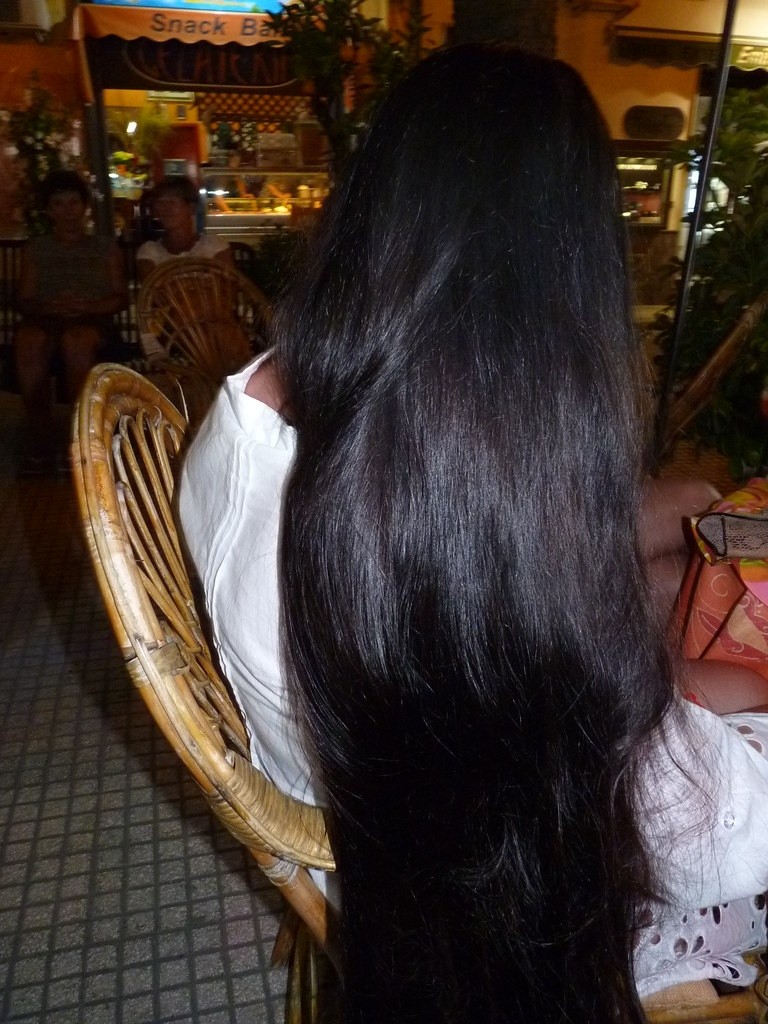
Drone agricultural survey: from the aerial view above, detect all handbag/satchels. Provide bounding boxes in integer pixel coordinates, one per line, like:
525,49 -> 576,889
695,513 -> 767,562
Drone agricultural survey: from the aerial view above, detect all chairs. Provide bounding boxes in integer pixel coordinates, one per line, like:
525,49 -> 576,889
138,258 -> 276,426
71,360 -> 768,1024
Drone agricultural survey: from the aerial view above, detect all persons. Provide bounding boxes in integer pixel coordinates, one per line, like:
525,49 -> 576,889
176,40 -> 767,1023
12,168 -> 127,478
136,175 -> 239,367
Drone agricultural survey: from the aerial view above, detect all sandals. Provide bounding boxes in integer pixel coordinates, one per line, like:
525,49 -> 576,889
57,453 -> 74,475
21,455 -> 43,475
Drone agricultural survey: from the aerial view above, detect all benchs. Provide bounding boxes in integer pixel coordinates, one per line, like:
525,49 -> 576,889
0,237 -> 269,394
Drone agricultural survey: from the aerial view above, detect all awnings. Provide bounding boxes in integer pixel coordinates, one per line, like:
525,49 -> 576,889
612,0 -> 768,73
68,0 -> 328,48
